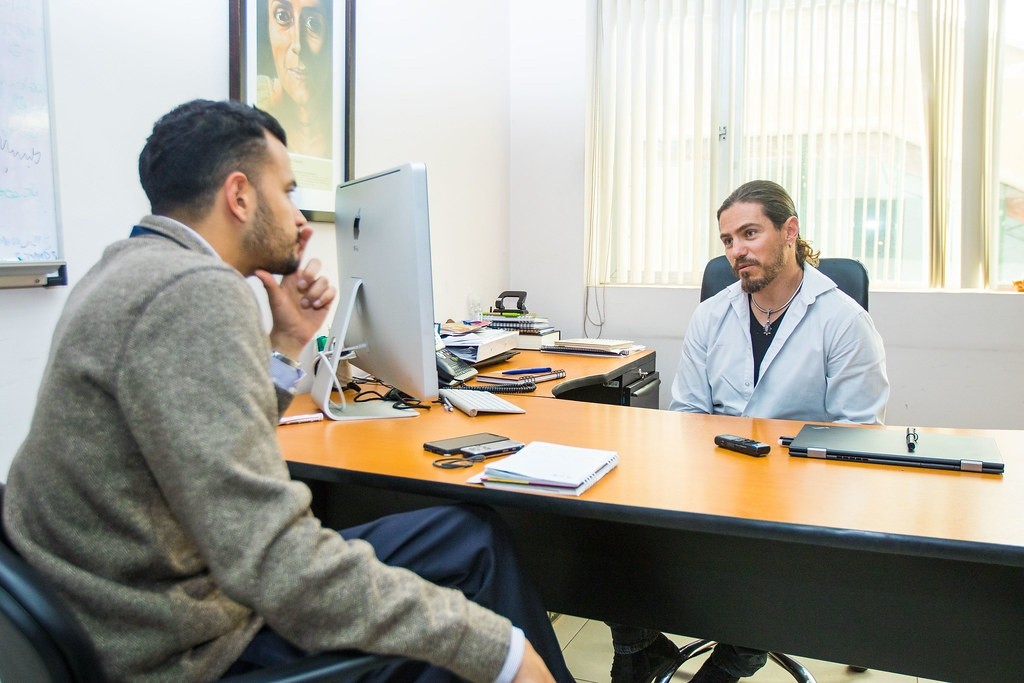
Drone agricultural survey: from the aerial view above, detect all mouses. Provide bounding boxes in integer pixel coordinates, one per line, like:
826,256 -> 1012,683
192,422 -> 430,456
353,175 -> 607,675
384,387 -> 413,400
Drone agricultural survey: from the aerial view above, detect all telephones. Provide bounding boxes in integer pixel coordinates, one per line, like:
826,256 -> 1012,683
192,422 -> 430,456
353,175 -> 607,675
435,349 -> 478,387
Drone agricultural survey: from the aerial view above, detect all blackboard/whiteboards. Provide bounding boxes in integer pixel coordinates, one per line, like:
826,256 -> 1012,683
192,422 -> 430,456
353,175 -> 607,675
0,0 -> 69,289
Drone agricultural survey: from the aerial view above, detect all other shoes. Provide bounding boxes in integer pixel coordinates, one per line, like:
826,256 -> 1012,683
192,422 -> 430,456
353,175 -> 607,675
610,634 -> 680,683
688,657 -> 740,683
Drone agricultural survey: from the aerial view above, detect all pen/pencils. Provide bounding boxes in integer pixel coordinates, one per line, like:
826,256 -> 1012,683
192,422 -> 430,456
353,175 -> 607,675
502,368 -> 551,374
906,428 -> 918,450
441,396 -> 453,412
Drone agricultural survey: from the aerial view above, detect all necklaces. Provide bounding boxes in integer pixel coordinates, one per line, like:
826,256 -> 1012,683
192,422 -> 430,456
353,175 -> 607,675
751,278 -> 803,336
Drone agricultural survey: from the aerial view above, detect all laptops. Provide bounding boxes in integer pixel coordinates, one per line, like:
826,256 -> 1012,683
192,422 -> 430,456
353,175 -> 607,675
789,424 -> 1005,474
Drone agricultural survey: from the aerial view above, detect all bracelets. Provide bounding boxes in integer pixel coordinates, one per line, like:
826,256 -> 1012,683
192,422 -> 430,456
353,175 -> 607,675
272,350 -> 297,367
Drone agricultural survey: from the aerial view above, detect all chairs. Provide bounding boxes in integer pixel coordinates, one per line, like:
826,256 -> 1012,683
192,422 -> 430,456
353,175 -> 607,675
0,483 -> 444,683
650,249 -> 870,683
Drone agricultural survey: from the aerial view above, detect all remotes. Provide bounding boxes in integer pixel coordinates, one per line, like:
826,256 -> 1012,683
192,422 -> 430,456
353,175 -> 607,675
713,434 -> 770,456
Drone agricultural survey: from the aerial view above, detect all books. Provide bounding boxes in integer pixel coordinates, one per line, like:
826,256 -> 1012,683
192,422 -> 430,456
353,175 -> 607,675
538,338 -> 634,355
476,369 -> 566,384
482,315 -> 554,334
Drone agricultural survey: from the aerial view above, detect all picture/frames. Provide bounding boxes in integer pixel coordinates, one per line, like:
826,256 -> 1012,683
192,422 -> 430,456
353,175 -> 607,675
228,0 -> 356,223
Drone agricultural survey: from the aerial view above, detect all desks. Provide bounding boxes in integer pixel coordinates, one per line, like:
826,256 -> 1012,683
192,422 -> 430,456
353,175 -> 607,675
276,345 -> 1024,683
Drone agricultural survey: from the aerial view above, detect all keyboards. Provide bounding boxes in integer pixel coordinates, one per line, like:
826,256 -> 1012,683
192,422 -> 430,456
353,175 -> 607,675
438,389 -> 527,417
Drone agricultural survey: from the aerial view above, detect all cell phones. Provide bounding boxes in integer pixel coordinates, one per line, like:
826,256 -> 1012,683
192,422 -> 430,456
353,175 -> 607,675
423,432 -> 509,456
459,440 -> 525,458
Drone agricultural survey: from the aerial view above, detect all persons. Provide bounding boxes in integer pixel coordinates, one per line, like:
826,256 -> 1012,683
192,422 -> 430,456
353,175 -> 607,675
603,180 -> 891,683
0,99 -> 573,683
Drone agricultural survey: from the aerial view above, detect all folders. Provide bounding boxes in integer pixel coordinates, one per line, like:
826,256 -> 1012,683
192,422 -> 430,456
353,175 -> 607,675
442,328 -> 519,363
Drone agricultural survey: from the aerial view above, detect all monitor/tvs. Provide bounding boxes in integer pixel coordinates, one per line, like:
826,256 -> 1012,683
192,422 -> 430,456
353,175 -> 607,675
311,162 -> 440,420
